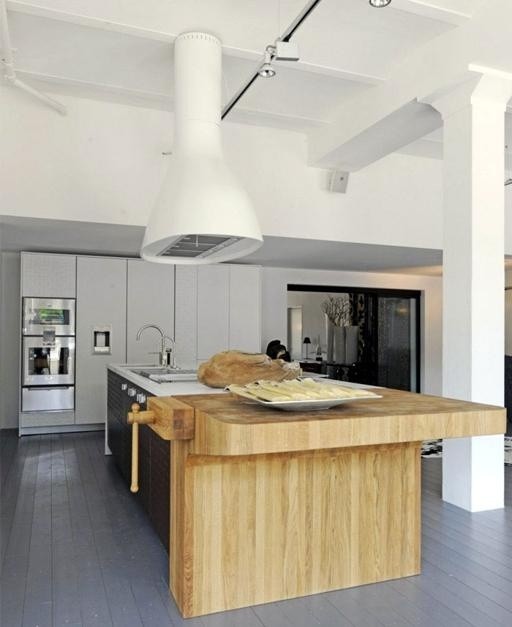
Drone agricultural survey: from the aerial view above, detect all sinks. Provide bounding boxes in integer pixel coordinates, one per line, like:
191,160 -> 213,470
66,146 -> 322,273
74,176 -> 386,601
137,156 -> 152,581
117,364 -> 159,368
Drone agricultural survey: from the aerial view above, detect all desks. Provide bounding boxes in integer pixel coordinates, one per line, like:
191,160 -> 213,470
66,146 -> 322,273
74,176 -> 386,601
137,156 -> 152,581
298,362 -> 322,373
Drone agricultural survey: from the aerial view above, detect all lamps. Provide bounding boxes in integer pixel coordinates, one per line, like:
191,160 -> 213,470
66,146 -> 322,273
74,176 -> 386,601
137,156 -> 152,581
257,50 -> 276,79
304,338 -> 311,358
371,0 -> 391,8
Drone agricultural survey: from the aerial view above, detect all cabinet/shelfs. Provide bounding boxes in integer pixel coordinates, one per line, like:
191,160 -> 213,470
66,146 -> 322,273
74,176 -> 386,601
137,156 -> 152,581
176,263 -> 229,369
231,265 -> 261,354
77,254 -> 127,432
127,257 -> 175,368
18,410 -> 77,435
19,251 -> 77,298
107,367 -> 170,557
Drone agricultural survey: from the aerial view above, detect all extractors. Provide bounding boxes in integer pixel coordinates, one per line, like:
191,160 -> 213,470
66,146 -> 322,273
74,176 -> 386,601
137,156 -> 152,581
139,34 -> 262,265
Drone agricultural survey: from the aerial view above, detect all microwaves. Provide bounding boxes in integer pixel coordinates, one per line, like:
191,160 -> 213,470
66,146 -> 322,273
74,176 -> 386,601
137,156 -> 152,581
21,297 -> 76,336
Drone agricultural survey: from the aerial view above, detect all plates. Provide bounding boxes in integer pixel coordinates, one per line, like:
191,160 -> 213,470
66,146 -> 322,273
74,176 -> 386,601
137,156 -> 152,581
228,381 -> 384,411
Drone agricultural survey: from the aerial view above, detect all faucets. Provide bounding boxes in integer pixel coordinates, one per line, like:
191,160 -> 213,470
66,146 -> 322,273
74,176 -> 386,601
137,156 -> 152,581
135,324 -> 165,368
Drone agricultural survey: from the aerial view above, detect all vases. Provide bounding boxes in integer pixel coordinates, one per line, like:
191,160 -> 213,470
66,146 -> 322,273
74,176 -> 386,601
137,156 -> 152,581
324,315 -> 357,365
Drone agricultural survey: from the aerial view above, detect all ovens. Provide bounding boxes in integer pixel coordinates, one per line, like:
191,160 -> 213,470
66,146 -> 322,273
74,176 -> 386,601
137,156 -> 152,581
22,338 -> 76,412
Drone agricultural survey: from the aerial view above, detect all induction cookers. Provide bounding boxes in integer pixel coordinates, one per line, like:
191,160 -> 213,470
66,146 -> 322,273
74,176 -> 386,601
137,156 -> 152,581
141,369 -> 198,384
